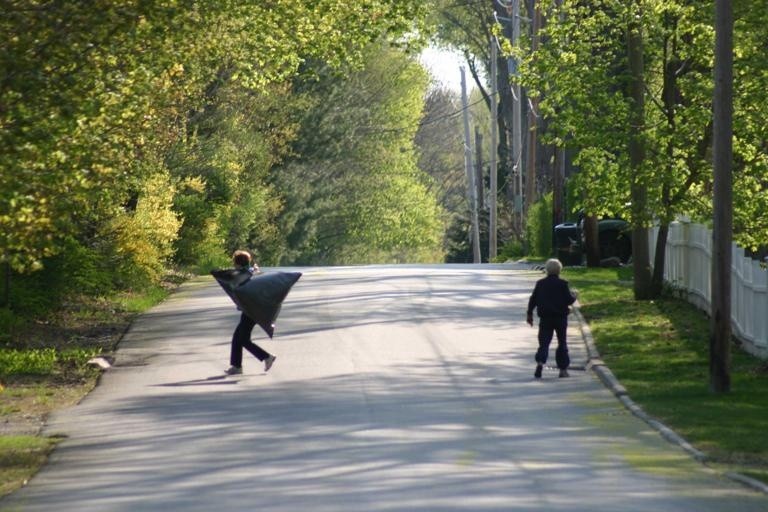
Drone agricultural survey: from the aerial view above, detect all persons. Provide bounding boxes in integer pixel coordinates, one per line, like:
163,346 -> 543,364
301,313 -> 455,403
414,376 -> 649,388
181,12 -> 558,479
210,249 -> 276,375
526,257 -> 579,378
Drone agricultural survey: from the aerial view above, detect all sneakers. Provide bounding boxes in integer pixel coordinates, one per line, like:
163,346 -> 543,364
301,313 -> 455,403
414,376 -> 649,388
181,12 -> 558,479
558,369 -> 571,378
224,365 -> 244,375
533,363 -> 542,378
263,354 -> 276,372
